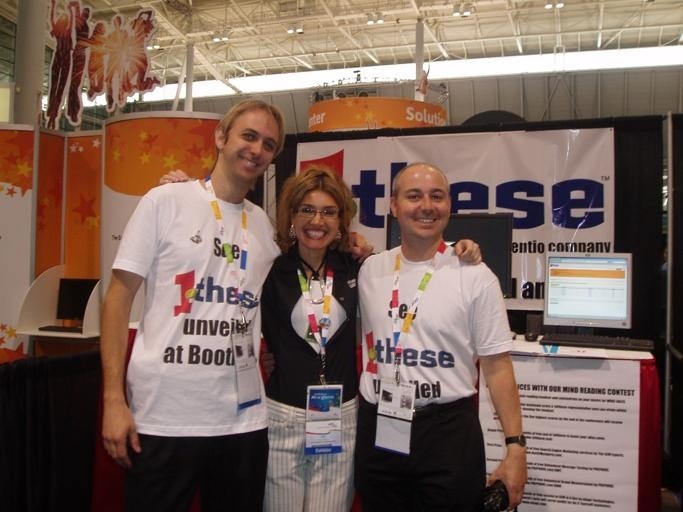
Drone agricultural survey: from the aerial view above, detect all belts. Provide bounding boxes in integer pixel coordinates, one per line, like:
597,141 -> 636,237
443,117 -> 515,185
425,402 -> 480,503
359,396 -> 476,420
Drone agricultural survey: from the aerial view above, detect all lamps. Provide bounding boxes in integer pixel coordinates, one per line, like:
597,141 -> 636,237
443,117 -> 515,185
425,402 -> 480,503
453,4 -> 461,17
297,20 -> 304,33
212,34 -> 221,43
462,2 -> 471,17
222,31 -> 228,41
555,0 -> 564,8
146,43 -> 152,49
544,1 -> 552,10
376,13 -> 383,24
287,24 -> 294,34
154,41 -> 160,49
366,14 -> 374,25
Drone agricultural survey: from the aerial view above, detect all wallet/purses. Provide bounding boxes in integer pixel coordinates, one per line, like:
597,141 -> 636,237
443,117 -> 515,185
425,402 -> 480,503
482,480 -> 518,512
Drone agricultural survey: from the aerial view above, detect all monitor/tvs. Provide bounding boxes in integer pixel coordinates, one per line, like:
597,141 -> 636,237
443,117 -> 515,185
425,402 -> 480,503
55,277 -> 100,320
541,252 -> 634,330
385,212 -> 516,299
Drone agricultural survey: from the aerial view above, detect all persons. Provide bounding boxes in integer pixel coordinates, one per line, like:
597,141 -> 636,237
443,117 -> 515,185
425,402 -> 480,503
85,23 -> 107,100
353,162 -> 528,511
45,0 -> 74,130
260,164 -> 483,512
99,98 -> 374,512
65,0 -> 90,123
124,10 -> 161,95
103,16 -> 129,111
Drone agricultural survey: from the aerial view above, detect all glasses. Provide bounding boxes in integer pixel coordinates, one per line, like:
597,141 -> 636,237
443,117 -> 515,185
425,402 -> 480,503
294,206 -> 341,219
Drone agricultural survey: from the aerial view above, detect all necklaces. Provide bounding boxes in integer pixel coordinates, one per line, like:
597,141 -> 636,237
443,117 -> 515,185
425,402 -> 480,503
300,258 -> 329,305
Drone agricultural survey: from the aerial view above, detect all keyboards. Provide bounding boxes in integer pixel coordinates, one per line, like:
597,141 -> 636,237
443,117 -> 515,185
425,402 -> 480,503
37,325 -> 86,334
538,330 -> 655,353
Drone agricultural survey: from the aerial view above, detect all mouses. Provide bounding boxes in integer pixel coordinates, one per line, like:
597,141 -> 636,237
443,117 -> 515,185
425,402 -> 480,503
522,326 -> 539,341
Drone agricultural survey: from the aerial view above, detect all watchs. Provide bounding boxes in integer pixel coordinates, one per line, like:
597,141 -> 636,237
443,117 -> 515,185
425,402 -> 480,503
503,433 -> 527,446
